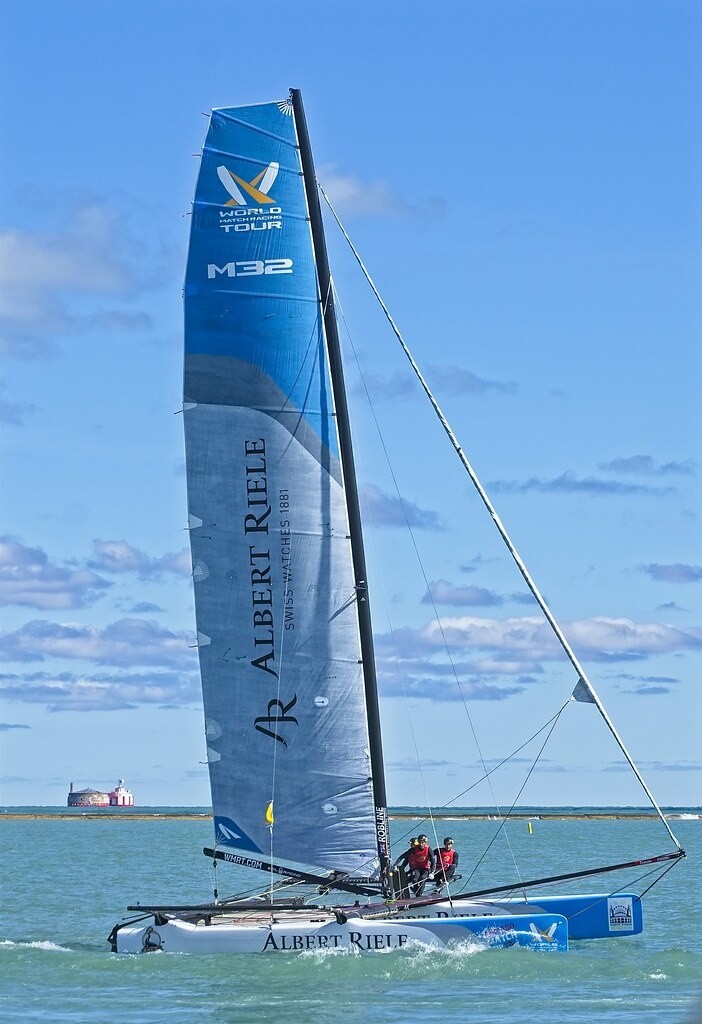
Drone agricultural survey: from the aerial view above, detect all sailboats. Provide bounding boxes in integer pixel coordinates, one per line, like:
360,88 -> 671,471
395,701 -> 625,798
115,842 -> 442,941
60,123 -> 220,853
108,87 -> 687,960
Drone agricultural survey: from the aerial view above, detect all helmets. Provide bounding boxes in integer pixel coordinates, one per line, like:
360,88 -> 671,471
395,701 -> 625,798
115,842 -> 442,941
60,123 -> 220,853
444,837 -> 454,845
408,838 -> 419,846
418,834 -> 428,843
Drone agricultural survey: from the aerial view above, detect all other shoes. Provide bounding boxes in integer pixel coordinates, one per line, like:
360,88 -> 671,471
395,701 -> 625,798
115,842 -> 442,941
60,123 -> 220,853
416,893 -> 422,897
410,887 -> 416,893
436,879 -> 442,887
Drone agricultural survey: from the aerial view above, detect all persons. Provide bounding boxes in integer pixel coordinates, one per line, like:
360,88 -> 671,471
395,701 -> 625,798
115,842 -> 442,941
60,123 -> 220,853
393,834 -> 459,897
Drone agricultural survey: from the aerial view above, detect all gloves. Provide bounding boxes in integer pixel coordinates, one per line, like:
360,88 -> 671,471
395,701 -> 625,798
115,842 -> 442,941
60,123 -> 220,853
444,863 -> 449,868
393,865 -> 397,872
429,873 -> 434,880
